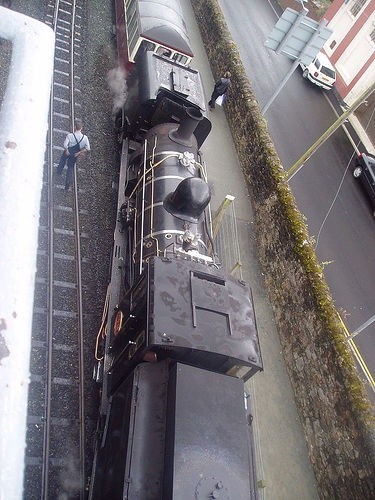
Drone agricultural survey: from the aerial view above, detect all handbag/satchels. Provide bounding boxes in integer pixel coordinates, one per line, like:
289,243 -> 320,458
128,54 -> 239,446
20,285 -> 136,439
215,94 -> 224,106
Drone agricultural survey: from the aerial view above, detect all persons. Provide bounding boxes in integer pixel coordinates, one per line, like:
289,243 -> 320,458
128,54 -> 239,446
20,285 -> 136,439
208,71 -> 231,112
56,121 -> 92,191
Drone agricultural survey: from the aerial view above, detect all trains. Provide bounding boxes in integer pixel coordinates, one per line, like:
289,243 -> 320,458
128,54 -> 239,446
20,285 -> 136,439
87,0 -> 265,500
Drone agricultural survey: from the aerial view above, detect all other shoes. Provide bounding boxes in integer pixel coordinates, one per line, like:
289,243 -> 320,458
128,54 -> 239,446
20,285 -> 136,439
65,187 -> 69,190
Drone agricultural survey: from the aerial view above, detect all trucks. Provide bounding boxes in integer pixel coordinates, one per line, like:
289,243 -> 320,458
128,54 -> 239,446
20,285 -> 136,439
299,51 -> 336,91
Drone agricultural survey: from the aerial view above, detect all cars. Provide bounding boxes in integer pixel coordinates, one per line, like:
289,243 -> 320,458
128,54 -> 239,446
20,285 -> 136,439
352,151 -> 375,219
